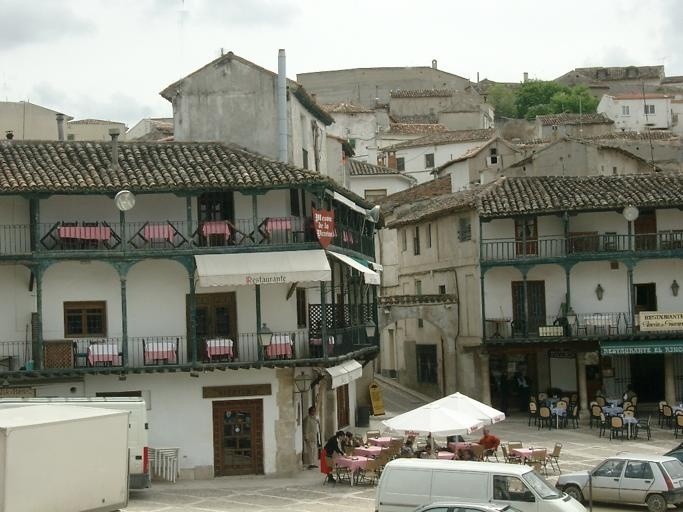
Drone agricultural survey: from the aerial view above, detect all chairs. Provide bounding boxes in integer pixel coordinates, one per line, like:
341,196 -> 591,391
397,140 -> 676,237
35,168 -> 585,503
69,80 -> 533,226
40,218 -> 306,249
529,392 -> 581,431
72,342 -> 88,368
576,313 -> 638,336
658,400 -> 683,438
321,430 -> 562,488
589,391 -> 651,440
511,320 -> 525,336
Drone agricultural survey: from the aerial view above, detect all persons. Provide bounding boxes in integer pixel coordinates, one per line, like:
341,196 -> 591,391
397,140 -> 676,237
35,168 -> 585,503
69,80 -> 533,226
303,407 -> 319,468
595,383 -> 607,398
480,427 -> 500,456
341,431 -> 353,453
426,432 -> 437,446
622,384 -> 637,402
422,445 -> 436,459
320,431 -> 346,483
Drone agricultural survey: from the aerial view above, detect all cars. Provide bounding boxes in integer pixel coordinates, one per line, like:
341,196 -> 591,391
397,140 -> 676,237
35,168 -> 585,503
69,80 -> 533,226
410,500 -> 524,512
555,452 -> 682,512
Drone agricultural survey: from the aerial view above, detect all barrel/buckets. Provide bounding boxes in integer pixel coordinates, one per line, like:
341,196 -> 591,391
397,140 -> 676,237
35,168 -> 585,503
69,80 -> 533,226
497,321 -> 512,338
357,405 -> 371,428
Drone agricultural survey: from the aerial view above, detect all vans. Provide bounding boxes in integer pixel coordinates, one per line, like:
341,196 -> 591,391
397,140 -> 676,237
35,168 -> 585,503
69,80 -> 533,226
374,458 -> 588,512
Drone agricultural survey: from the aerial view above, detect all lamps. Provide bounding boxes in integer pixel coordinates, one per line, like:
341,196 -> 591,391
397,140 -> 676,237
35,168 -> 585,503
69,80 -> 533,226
259,322 -> 273,345
114,189 -> 137,211
671,280 -> 680,296
567,308 -> 576,324
595,284 -> 604,300
623,206 -> 639,221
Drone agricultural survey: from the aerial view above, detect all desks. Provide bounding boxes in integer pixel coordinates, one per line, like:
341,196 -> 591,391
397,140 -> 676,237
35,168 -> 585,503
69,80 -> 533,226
86,336 -> 335,367
485,320 -> 510,339
